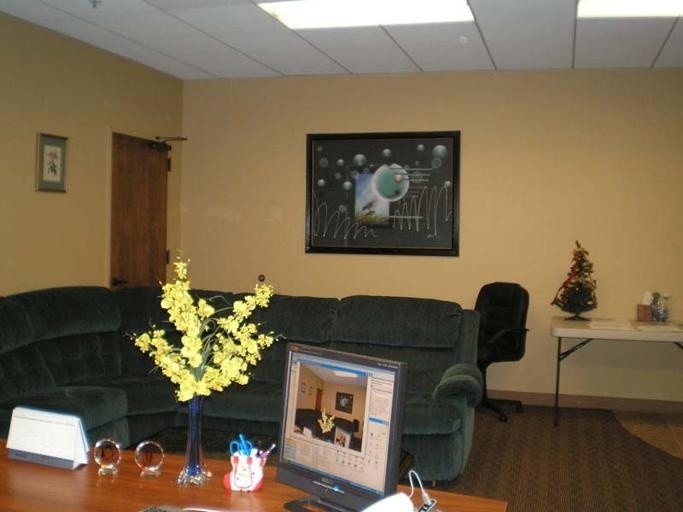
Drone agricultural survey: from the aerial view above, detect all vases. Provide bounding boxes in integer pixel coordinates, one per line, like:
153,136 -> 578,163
177,390 -> 212,486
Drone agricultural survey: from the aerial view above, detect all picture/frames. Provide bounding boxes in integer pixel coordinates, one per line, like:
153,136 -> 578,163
32,131 -> 69,193
304,131 -> 463,260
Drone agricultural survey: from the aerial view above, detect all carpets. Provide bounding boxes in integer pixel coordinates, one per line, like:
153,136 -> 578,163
454,398 -> 680,511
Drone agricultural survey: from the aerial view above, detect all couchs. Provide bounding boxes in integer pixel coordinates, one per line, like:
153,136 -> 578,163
1,285 -> 486,488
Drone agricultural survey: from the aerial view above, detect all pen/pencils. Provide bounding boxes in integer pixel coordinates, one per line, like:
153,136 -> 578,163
239,433 -> 277,458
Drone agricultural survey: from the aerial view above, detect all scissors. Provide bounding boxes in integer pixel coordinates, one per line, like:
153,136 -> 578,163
229,440 -> 252,457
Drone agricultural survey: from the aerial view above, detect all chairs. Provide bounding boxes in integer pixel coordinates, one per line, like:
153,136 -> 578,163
475,279 -> 531,423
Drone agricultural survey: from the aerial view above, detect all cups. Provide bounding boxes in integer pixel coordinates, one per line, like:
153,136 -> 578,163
637,304 -> 653,322
229,448 -> 265,492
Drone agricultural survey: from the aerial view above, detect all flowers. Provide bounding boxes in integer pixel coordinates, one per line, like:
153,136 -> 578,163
121,245 -> 288,407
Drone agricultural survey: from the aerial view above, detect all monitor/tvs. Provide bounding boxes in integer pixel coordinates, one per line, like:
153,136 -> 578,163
274,343 -> 408,512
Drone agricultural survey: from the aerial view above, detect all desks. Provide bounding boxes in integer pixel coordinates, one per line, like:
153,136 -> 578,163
550,315 -> 683,429
3,437 -> 510,512
